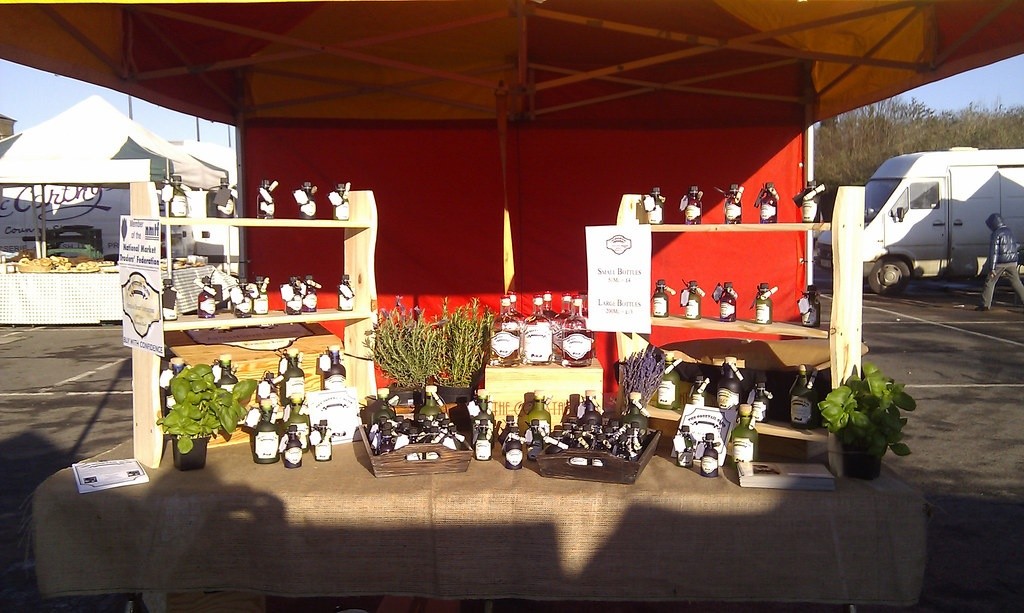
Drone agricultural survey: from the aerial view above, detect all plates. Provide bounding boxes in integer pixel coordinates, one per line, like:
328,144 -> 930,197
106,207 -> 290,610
0,256 -> 208,273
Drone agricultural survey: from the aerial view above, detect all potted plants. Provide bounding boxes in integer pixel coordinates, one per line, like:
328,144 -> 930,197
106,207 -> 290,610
157,365 -> 257,471
815,360 -> 917,479
362,299 -> 495,408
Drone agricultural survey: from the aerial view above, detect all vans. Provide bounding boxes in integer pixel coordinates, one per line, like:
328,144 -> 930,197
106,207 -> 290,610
811,150 -> 1023,295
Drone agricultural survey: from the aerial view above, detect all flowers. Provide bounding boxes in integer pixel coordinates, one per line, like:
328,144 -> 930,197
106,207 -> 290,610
619,345 -> 668,415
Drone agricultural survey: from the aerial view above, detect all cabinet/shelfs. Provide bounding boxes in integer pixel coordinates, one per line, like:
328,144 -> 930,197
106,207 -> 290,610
619,186 -> 865,450
126,180 -> 378,467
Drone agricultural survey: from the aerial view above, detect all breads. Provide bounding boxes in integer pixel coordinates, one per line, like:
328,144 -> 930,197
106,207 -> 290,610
20,256 -> 99,272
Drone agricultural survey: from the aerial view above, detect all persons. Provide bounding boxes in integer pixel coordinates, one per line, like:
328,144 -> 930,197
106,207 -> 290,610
975,212 -> 1024,311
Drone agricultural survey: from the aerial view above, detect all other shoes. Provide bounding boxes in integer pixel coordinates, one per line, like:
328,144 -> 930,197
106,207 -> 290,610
975,305 -> 989,311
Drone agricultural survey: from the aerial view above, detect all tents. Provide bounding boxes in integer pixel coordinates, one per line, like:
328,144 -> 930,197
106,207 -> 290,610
0,94 -> 233,310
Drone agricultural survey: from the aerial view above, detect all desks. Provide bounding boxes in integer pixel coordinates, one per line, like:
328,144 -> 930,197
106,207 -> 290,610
1,266 -> 227,326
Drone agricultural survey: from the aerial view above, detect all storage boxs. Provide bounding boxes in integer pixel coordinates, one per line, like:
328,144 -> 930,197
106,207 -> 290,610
484,352 -> 603,435
358,426 -> 475,478
536,429 -> 660,484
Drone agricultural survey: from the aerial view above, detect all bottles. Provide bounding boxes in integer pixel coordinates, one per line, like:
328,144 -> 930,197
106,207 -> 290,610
652,279 -> 669,317
257,180 -> 275,219
363,385 -> 647,471
164,345 -> 347,469
648,187 -> 664,224
168,175 -> 186,217
489,289 -> 594,368
298,182 -> 316,220
801,285 -> 820,327
756,282 -> 772,324
685,281 -> 702,319
215,177 -> 234,218
719,282 -> 736,322
725,184 -> 742,224
685,186 -> 702,224
802,180 -> 821,222
332,184 -> 350,220
163,275 -> 355,322
662,351 -> 816,473
760,182 -> 778,223
